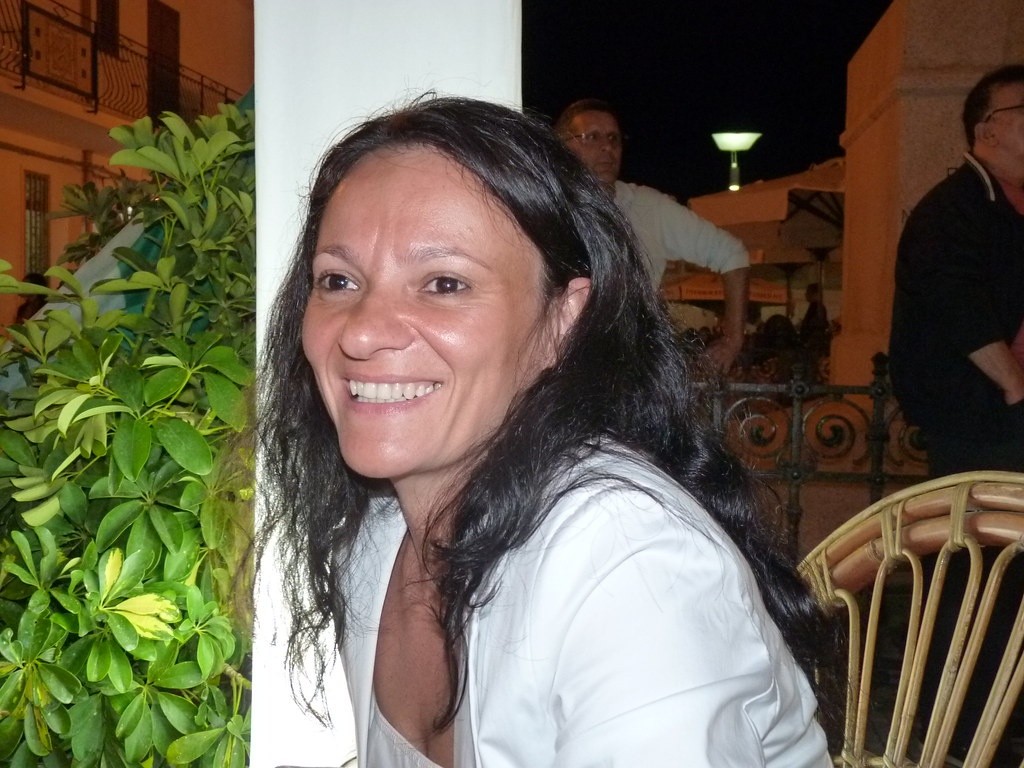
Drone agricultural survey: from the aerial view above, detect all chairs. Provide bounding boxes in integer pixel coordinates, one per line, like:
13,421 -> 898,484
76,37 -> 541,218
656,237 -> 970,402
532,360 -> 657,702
795,470 -> 1024,768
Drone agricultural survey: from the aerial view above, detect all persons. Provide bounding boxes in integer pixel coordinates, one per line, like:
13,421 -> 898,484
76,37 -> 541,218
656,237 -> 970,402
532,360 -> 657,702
556,97 -> 751,386
889,59 -> 1024,768
684,282 -> 829,368
15,273 -> 49,324
248,89 -> 834,767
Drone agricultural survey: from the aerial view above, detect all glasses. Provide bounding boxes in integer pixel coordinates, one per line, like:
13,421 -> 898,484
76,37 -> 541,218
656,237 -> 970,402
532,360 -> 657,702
565,131 -> 629,149
